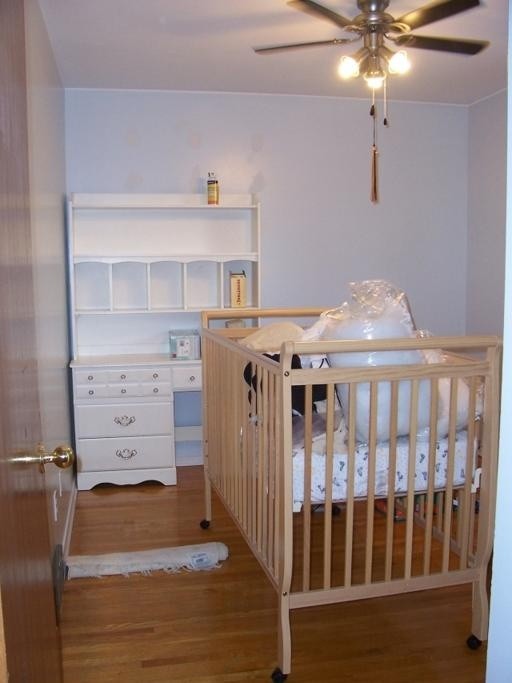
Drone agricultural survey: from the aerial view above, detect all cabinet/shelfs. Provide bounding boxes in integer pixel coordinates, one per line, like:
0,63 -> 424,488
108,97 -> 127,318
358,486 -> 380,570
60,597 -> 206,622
67,192 -> 262,491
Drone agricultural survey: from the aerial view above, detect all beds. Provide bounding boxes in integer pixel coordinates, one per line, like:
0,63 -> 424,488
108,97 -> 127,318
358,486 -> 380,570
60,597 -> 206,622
201,307 -> 504,683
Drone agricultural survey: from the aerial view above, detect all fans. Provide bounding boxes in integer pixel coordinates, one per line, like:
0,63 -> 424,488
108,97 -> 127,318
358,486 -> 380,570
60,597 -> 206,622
254,0 -> 490,56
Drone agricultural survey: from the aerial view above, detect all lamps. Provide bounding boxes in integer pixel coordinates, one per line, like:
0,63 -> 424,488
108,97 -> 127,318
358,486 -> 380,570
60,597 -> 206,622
337,30 -> 410,90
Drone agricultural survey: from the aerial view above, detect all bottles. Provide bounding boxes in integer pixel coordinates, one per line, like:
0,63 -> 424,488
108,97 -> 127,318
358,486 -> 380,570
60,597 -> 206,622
207,172 -> 218,204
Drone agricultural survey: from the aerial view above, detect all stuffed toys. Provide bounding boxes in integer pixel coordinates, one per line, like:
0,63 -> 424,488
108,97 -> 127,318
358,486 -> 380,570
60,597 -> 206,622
240,350 -> 334,421
